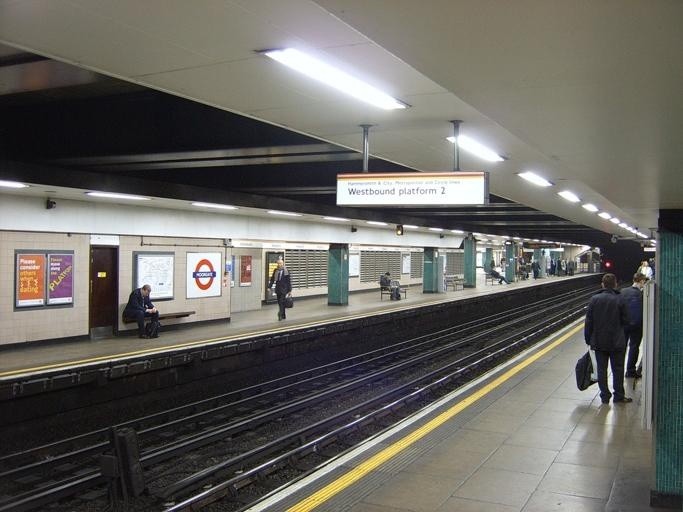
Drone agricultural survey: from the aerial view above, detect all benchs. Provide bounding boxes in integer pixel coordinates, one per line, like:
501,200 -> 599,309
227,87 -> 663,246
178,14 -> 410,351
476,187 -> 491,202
483,274 -> 499,286
122,310 -> 196,338
443,275 -> 467,291
379,280 -> 408,300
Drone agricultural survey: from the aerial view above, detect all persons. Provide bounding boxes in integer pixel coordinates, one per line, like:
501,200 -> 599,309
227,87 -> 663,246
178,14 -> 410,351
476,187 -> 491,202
120,284 -> 160,340
378,270 -> 402,302
640,261 -> 652,283
487,254 -> 575,285
649,258 -> 655,276
264,258 -> 293,323
636,261 -> 644,274
584,273 -> 633,404
619,271 -> 646,379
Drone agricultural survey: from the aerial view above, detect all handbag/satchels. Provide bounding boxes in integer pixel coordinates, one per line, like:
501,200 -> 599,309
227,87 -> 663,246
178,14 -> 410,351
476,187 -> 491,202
574,351 -> 597,390
283,298 -> 293,308
146,321 -> 162,339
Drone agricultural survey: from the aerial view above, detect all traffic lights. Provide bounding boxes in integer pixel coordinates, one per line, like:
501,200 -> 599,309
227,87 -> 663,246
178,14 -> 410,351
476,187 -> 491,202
606,261 -> 612,269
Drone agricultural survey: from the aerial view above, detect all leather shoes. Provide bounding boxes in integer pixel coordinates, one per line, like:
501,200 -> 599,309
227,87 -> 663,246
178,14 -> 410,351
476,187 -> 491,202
625,371 -> 641,379
602,399 -> 609,404
613,396 -> 632,402
